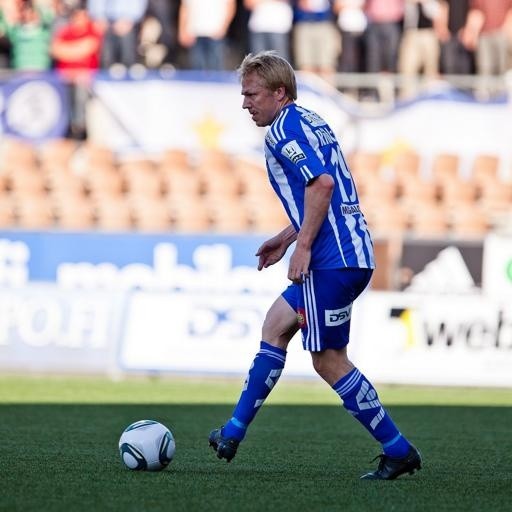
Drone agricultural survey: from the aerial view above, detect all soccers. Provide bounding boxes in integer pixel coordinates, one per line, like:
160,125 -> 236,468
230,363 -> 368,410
118,420 -> 175,472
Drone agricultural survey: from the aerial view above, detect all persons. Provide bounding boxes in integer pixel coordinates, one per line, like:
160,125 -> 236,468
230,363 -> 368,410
209,50 -> 423,480
0,0 -> 510,145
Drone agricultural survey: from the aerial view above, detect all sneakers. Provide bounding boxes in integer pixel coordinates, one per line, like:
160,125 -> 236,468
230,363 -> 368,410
208,425 -> 240,464
359,444 -> 424,482
